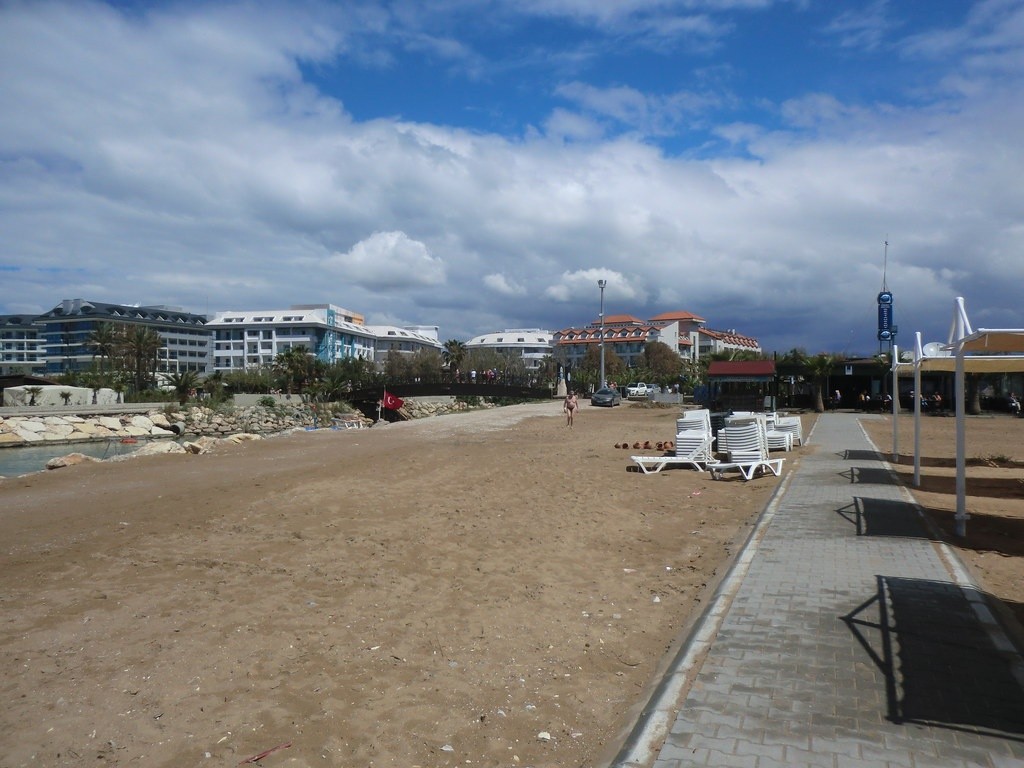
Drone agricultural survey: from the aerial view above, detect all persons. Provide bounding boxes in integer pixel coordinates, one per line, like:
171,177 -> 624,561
614,382 -> 618,387
830,390 -> 1024,416
564,390 -> 579,429
454,366 -> 536,387
608,381 -> 614,388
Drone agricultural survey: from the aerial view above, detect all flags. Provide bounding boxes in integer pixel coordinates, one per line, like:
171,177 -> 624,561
383,390 -> 404,410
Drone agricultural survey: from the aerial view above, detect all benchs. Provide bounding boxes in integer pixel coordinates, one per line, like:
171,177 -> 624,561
631,409 -> 805,480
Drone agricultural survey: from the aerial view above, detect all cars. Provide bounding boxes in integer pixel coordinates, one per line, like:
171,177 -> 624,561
626,382 -> 661,396
591,388 -> 622,407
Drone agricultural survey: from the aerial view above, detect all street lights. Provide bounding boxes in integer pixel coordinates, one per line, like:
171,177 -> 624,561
597,279 -> 607,390
